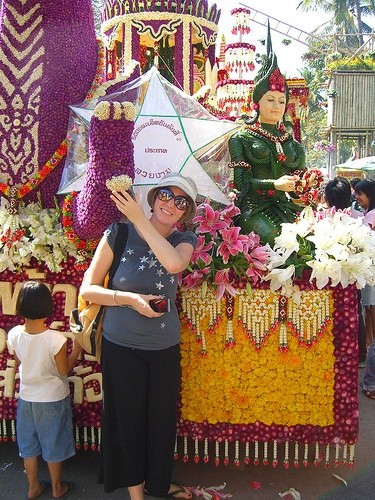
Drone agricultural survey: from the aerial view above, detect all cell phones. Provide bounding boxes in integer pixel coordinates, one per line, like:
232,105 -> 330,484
149,298 -> 170,314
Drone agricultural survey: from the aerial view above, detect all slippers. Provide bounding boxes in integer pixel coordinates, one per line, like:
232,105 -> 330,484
143,482 -> 192,500
359,381 -> 375,399
26,480 -> 51,500
52,481 -> 75,500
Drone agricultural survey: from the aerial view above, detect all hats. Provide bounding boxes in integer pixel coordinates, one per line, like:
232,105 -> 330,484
146,172 -> 198,222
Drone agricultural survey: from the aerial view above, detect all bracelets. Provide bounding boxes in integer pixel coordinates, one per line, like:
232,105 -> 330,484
114,290 -> 128,307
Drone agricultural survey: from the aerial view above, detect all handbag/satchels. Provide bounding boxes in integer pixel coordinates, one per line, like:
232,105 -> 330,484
69,223 -> 128,357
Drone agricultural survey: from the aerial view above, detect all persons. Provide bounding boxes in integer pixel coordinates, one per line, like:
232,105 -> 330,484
229,53 -> 305,245
319,177 -> 375,401
81,172 -> 198,500
6,280 -> 83,500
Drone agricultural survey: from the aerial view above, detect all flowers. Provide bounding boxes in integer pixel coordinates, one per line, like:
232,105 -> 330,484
0,169 -> 375,304
313,139 -> 335,153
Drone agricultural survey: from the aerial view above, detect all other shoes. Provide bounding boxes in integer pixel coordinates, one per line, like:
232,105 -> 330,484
359,348 -> 369,367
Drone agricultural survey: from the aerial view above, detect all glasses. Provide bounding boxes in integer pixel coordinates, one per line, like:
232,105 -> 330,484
156,186 -> 191,211
